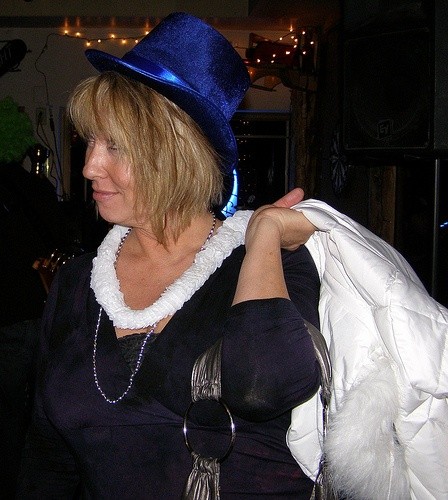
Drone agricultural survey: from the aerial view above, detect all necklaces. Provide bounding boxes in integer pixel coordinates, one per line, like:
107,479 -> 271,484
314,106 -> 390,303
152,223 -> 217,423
90,208 -> 253,329
93,204 -> 216,403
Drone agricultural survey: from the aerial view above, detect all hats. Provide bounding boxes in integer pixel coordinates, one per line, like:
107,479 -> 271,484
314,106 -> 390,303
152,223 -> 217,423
82,11 -> 253,175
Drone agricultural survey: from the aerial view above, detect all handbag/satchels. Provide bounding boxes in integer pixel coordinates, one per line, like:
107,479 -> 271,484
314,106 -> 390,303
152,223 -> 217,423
183,317 -> 354,500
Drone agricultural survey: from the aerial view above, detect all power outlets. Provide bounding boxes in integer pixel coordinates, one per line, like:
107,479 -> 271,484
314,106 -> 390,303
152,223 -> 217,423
36,107 -> 47,125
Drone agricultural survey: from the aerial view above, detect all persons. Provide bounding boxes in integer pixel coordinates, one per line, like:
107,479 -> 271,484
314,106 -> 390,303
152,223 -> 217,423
29,11 -> 320,500
0,98 -> 61,500
